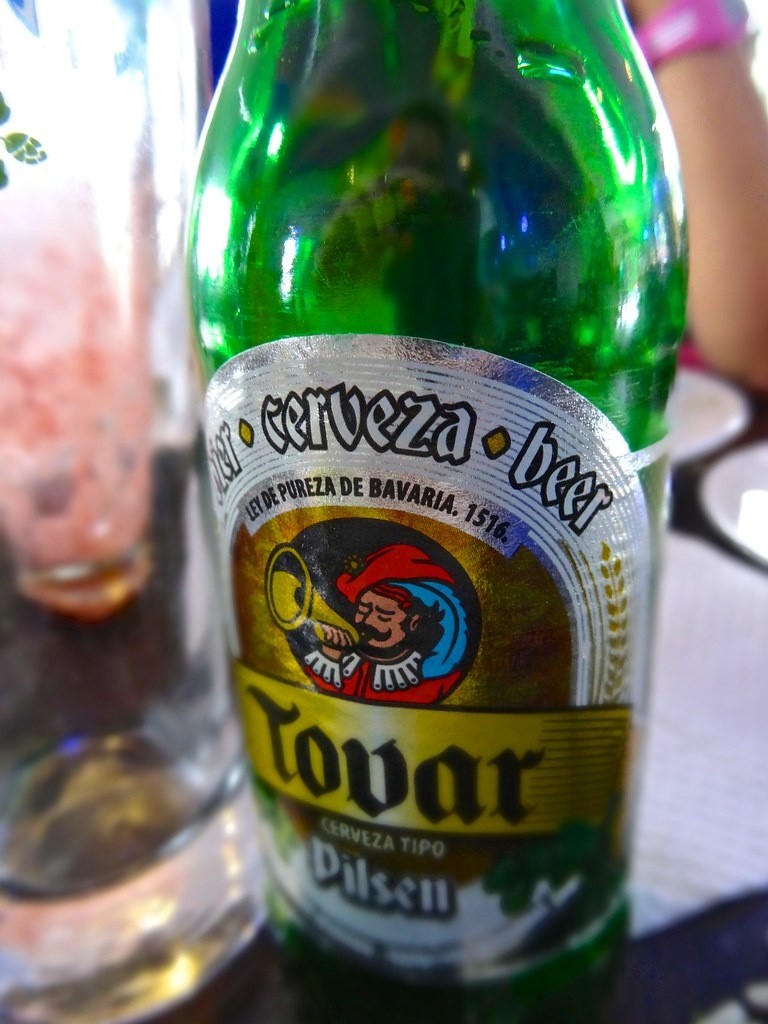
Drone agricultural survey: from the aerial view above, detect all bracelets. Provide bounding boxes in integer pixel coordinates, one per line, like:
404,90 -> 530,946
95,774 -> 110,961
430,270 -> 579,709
636,0 -> 748,68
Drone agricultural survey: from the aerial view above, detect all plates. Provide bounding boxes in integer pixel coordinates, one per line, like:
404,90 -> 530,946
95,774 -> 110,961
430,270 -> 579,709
664,368 -> 746,467
696,441 -> 768,567
627,532 -> 768,944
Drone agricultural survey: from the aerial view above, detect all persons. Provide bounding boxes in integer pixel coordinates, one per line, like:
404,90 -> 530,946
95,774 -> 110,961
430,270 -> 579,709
622,0 -> 768,385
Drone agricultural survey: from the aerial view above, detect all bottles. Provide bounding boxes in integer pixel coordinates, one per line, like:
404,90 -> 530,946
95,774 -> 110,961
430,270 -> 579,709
184,0 -> 692,1024
0,0 -> 274,1024
